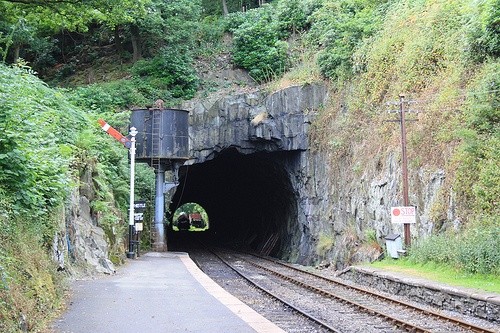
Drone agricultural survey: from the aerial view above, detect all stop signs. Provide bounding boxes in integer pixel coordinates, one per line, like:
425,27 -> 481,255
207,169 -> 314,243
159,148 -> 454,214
390,206 -> 416,224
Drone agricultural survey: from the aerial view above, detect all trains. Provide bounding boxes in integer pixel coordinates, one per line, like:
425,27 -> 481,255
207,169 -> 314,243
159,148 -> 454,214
176,212 -> 190,230
189,213 -> 204,228
